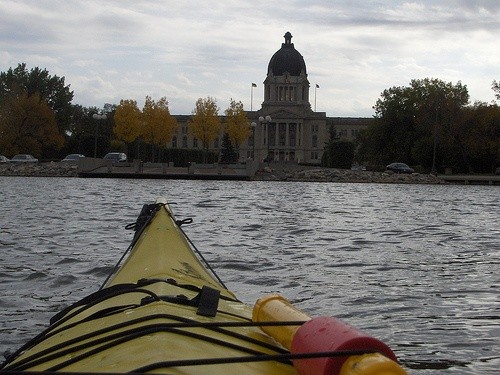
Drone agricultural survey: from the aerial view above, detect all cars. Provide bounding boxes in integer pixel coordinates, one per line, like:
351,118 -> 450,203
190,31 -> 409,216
386,163 -> 415,175
0,155 -> 10,164
61,154 -> 85,163
7,155 -> 38,165
103,152 -> 129,167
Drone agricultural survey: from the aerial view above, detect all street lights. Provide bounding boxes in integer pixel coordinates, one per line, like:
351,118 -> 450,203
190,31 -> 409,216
431,104 -> 447,175
248,122 -> 256,160
259,115 -> 271,167
92,114 -> 107,158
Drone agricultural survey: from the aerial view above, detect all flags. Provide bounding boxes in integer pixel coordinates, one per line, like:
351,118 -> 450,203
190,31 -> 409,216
316,85 -> 319,88
252,83 -> 256,87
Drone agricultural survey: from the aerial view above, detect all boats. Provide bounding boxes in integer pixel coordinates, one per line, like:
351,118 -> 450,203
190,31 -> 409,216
1,194 -> 402,370
